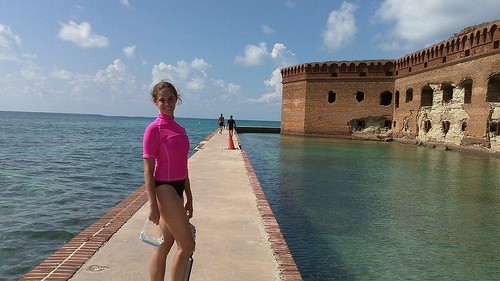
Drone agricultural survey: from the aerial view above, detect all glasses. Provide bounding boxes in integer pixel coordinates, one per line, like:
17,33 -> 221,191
140,220 -> 164,246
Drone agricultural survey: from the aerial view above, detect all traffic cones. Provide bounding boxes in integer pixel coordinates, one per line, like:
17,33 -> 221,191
228,132 -> 236,151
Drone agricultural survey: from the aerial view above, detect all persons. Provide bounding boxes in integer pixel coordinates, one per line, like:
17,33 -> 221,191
218,113 -> 224,134
143,82 -> 194,281
227,116 -> 236,137
